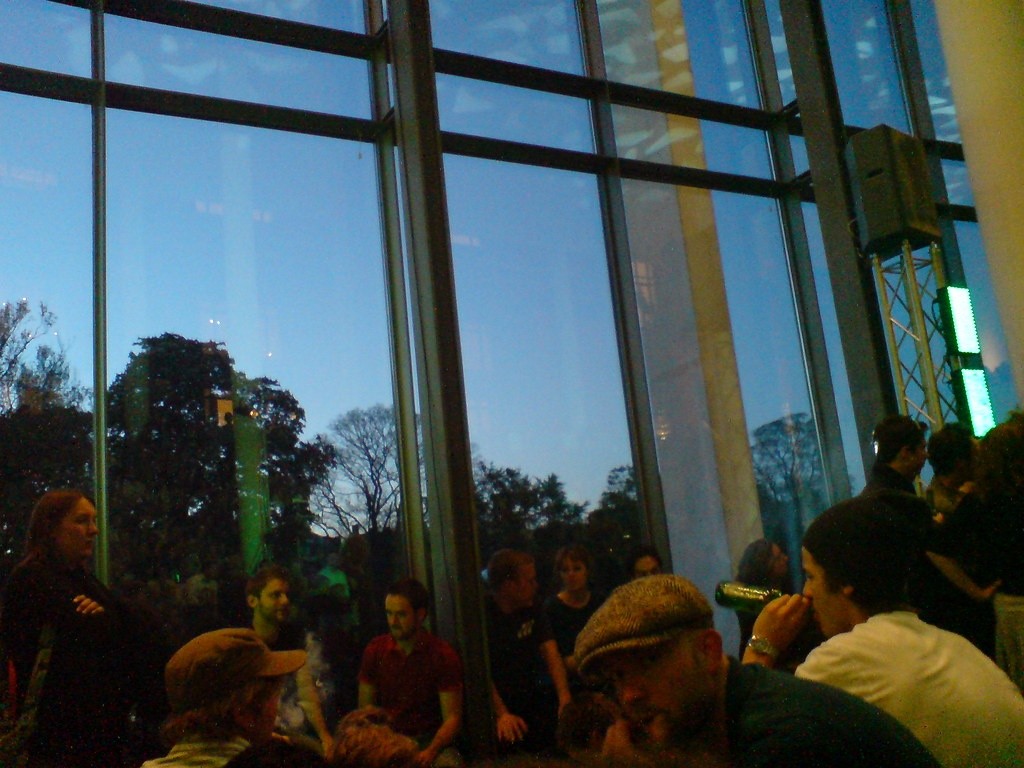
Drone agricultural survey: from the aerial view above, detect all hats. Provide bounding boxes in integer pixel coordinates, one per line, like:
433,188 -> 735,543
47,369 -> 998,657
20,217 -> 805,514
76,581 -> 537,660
801,489 -> 933,604
574,574 -> 713,677
164,628 -> 308,700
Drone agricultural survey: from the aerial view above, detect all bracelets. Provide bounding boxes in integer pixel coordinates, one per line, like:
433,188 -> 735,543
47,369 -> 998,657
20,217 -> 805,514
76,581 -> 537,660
746,637 -> 779,659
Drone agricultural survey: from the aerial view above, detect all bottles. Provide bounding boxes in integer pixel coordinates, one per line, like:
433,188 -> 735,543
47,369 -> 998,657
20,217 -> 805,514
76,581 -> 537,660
714,580 -> 814,615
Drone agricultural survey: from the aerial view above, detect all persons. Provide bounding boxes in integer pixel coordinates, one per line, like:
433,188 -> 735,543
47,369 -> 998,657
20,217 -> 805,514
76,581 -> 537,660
732,539 -> 801,674
0,490 -> 161,768
145,557 -> 218,617
138,628 -> 332,768
489,550 -> 571,753
543,546 -> 618,709
358,579 -> 465,768
230,569 -> 337,762
859,411 -> 1024,696
575,573 -> 944,768
741,498 -> 1024,768
625,544 -> 663,580
